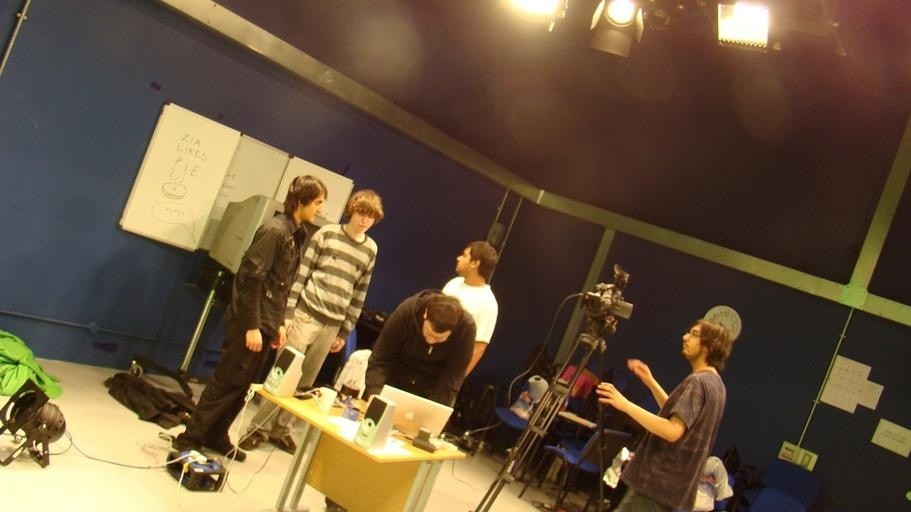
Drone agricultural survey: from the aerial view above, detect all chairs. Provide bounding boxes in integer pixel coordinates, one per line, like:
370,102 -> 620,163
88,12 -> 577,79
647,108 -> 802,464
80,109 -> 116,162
744,457 -> 822,509
518,429 -> 635,511
561,440 -> 592,456
332,326 -> 359,384
494,380 -> 582,434
746,487 -> 805,512
711,474 -> 738,509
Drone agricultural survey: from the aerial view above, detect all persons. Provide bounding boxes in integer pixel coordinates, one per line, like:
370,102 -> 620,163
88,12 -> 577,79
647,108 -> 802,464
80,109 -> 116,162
170,172 -> 330,465
440,239 -> 500,376
234,188 -> 386,451
593,317 -> 733,511
364,287 -> 477,411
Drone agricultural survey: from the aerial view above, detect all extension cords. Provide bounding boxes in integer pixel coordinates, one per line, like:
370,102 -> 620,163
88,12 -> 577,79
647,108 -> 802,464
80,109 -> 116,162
446,433 -> 475,452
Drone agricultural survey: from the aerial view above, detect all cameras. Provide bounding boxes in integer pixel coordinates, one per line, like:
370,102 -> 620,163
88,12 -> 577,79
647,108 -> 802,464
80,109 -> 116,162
581,264 -> 633,323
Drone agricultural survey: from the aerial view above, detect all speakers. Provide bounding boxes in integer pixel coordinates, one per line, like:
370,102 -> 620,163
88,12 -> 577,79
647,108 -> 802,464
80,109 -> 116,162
354,394 -> 395,451
263,346 -> 305,397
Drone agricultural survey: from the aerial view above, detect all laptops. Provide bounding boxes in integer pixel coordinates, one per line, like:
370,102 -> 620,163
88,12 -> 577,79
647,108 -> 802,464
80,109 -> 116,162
378,384 -> 454,439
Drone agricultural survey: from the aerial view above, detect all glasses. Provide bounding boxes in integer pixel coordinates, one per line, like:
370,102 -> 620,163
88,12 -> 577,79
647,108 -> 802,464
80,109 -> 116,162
686,330 -> 705,339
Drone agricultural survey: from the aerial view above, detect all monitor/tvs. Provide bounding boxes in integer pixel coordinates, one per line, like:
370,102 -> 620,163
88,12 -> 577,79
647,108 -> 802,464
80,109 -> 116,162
209,193 -> 337,278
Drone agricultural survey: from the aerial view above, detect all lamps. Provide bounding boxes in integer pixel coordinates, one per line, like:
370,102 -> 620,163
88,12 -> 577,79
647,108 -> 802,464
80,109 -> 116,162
497,0 -> 862,65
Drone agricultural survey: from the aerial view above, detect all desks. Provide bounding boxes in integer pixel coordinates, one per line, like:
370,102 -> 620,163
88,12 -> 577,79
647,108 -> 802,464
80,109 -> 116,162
250,383 -> 466,512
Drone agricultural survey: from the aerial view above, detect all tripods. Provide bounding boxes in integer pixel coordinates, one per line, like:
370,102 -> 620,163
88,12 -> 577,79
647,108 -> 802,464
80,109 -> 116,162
472,332 -> 605,512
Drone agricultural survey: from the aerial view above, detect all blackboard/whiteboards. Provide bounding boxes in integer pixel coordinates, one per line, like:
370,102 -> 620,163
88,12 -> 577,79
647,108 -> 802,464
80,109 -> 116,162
118,100 -> 356,252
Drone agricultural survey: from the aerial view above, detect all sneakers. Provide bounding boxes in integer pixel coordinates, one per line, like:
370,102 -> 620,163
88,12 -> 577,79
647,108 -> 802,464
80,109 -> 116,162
237,425 -> 267,451
171,434 -> 213,460
207,430 -> 247,463
269,429 -> 297,456
